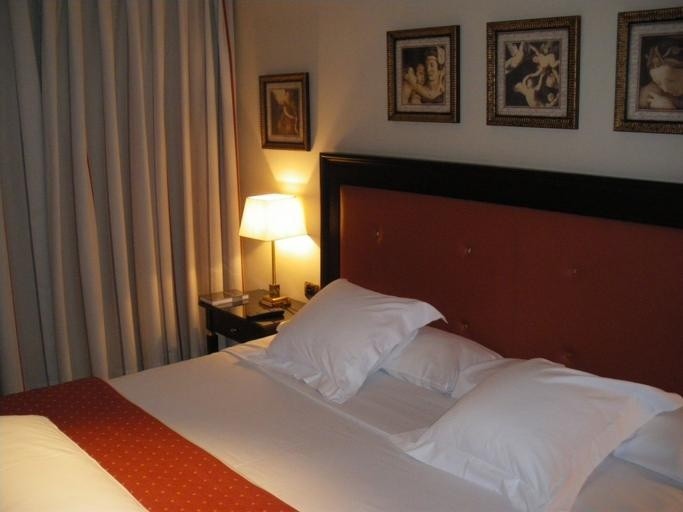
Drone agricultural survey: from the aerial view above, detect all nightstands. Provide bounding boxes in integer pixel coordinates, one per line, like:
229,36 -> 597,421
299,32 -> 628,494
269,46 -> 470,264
197,289 -> 306,355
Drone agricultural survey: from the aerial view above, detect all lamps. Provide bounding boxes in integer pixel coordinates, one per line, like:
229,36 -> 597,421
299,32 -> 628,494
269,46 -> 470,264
239,193 -> 306,308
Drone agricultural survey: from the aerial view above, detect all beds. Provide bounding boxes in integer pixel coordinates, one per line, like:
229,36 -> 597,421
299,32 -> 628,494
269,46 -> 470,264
2,152 -> 682,512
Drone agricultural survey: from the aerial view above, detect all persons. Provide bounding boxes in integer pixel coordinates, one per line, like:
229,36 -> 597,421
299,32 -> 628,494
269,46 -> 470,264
504,40 -> 561,108
638,46 -> 683,110
401,46 -> 445,105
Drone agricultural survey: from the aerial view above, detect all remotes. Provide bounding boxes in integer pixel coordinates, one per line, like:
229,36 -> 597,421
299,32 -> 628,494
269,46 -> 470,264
246,307 -> 284,321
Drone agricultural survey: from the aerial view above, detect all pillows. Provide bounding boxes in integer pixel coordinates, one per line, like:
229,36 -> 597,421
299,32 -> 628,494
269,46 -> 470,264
267,277 -> 448,404
386,325 -> 500,399
614,409 -> 680,484
404,359 -> 681,512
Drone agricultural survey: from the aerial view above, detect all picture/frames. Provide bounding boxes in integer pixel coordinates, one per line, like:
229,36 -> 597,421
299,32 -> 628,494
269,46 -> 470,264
386,25 -> 459,123
259,73 -> 310,151
613,8 -> 683,134
486,16 -> 579,128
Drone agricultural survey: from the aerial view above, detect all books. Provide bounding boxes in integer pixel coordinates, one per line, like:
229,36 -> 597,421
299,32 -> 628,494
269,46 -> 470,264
199,289 -> 249,307
